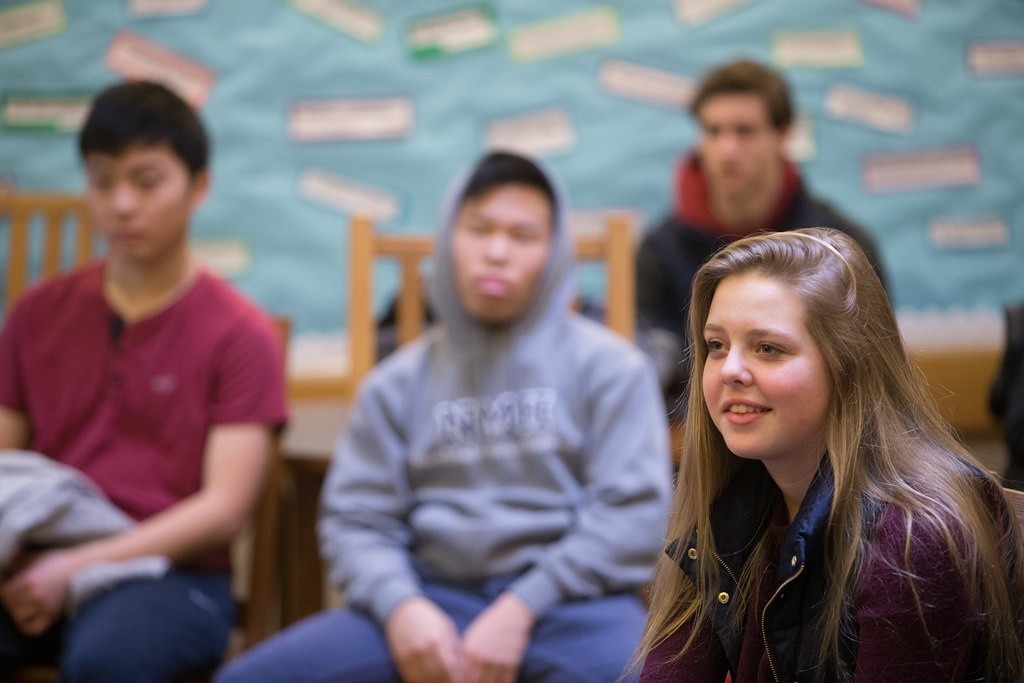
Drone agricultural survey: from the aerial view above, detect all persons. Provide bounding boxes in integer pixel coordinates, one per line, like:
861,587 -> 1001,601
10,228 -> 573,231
634,63 -> 885,458
217,148 -> 674,683
0,82 -> 291,683
615,228 -> 1024,682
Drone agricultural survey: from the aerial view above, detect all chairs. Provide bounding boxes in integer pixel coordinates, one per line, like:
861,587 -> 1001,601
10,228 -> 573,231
0,192 -> 97,313
346,210 -> 638,402
